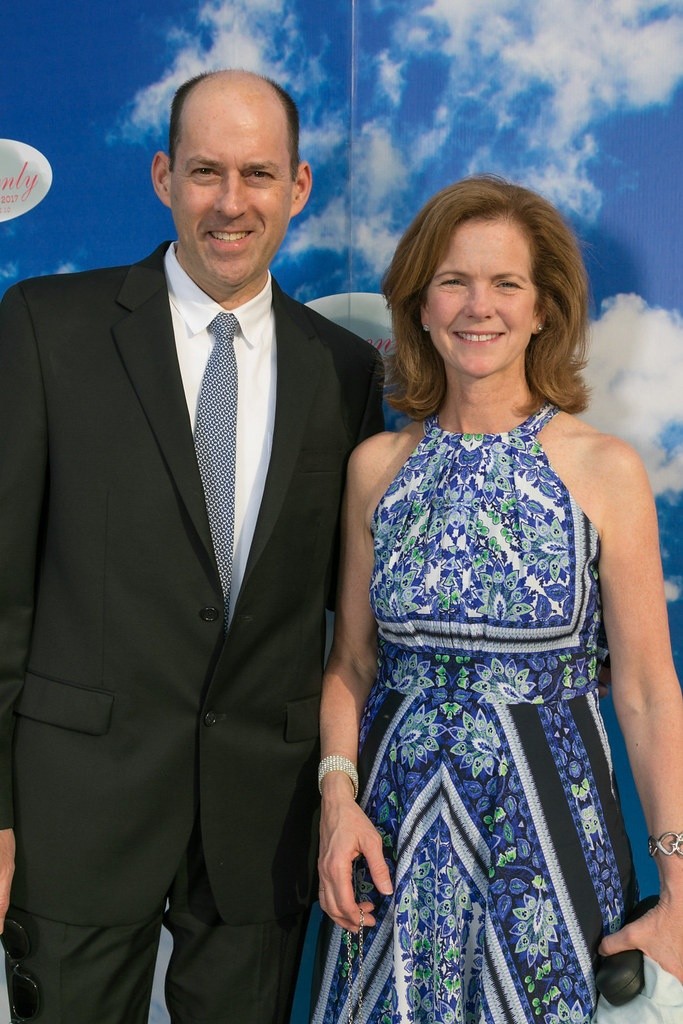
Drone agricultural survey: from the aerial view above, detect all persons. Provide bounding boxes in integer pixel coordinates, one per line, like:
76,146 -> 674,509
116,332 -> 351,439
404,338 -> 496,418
1,69 -> 386,1024
291,179 -> 683,1024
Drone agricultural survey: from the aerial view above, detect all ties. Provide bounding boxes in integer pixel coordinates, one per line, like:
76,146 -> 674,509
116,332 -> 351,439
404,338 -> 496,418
193,313 -> 241,646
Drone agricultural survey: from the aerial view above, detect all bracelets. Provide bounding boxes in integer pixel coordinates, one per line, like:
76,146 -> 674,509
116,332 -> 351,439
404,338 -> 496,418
647,832 -> 683,858
318,756 -> 358,800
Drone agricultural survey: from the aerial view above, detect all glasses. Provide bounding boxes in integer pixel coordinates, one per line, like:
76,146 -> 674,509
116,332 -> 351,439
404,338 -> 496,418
0,919 -> 40,1023
